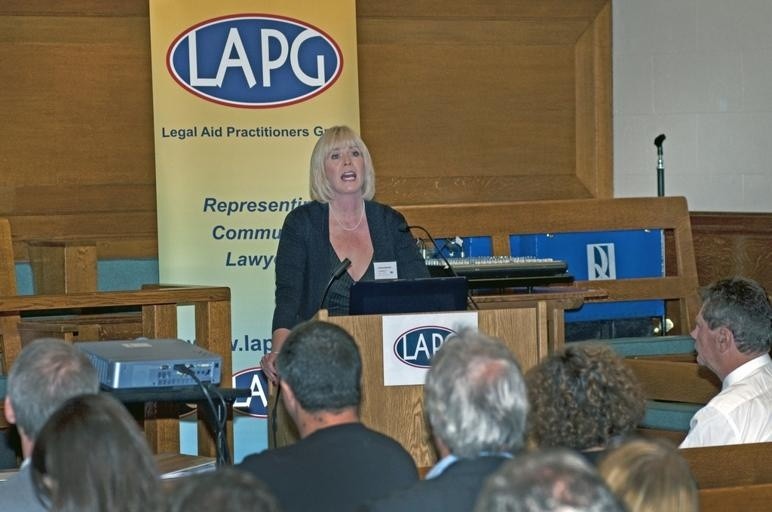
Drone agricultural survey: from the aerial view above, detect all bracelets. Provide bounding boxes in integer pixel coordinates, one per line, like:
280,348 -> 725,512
271,349 -> 280,355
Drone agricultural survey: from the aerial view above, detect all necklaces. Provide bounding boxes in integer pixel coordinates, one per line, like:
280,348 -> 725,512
328,195 -> 366,232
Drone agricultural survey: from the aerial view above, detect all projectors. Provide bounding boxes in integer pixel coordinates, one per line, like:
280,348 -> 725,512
73,336 -> 223,391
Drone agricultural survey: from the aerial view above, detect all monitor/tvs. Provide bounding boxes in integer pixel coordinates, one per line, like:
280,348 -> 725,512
349,276 -> 467,314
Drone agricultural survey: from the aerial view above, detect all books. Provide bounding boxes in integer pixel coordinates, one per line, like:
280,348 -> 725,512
158,452 -> 217,479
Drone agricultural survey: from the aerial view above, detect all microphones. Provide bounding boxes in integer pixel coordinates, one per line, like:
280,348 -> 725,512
318,258 -> 351,310
398,224 -> 480,310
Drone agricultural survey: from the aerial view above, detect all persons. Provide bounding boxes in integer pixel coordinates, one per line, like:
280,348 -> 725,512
361,325 -> 532,511
674,274 -> 772,450
170,463 -> 281,512
29,391 -> 169,511
258,125 -> 434,389
1,335 -> 99,511
470,443 -> 626,512
519,339 -> 650,452
235,319 -> 420,511
599,436 -> 702,512
